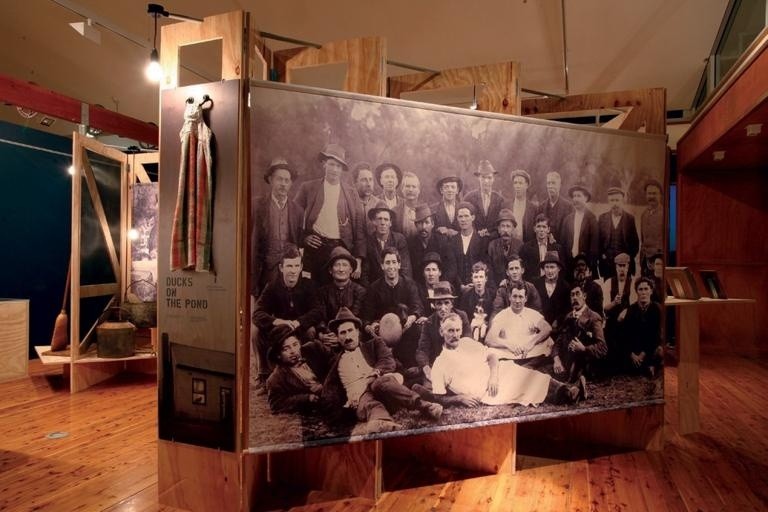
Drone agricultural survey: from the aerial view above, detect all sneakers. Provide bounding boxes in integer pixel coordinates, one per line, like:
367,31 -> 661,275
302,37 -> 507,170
367,420 -> 401,432
567,376 -> 588,405
426,403 -> 442,418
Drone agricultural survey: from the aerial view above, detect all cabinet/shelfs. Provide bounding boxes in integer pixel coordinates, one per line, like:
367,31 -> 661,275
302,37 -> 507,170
0,297 -> 31,385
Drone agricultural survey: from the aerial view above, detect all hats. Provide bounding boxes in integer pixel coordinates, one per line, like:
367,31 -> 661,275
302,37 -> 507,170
493,208 -> 518,227
428,282 -> 457,301
569,180 -> 664,203
375,160 -> 402,189
328,307 -> 362,330
415,205 -> 435,224
614,254 -> 630,266
269,324 -> 298,351
264,157 -> 297,182
368,202 -> 398,223
421,251 -> 441,266
540,250 -> 566,268
437,160 -> 532,193
322,246 -> 357,274
320,143 -> 349,173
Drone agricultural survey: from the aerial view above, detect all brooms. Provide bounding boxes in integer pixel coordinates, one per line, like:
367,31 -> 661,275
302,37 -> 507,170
50,263 -> 69,353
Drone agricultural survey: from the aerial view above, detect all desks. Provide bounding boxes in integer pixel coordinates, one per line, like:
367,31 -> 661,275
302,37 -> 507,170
662,294 -> 756,440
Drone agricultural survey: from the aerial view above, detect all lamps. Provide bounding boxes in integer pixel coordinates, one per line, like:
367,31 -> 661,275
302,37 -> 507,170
145,2 -> 203,83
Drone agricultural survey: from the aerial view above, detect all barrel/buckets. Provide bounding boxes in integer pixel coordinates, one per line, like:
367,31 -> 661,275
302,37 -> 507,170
94,305 -> 136,358
125,279 -> 158,329
